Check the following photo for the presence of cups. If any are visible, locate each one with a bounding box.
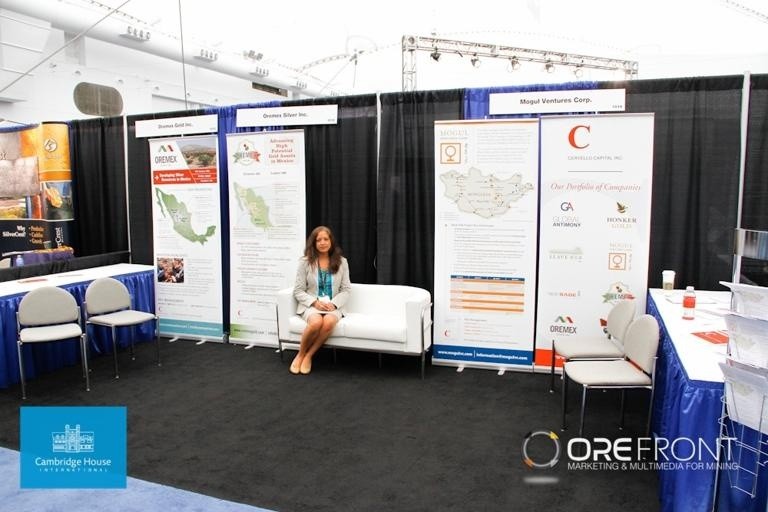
[662,270,676,296]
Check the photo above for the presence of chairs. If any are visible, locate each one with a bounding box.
[81,277,160,380]
[16,285,90,400]
[560,314,660,461]
[548,301,636,414]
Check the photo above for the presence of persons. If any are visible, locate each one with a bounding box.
[157,256,185,283]
[287,226,353,375]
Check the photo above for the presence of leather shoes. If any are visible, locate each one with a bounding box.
[300,356,312,375]
[289,353,301,375]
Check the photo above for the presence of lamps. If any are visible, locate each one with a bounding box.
[429,47,584,79]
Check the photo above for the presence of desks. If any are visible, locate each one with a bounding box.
[648,288,768,512]
[0,447,273,512]
[0,263,155,393]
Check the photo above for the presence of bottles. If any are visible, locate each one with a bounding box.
[682,285,697,320]
[16,254,24,266]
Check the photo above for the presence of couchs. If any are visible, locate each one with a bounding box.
[274,281,433,380]
